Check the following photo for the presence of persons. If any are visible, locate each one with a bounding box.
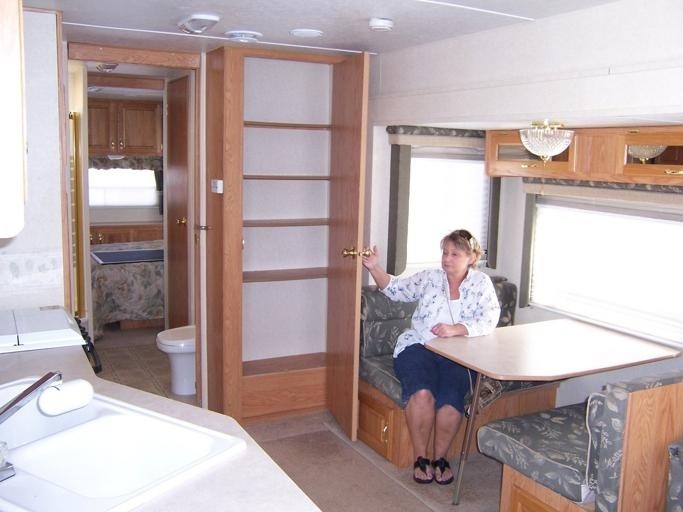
[362,229,501,485]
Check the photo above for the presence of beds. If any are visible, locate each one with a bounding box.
[91,240,167,329]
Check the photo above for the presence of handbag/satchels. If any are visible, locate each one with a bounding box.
[463,377,504,418]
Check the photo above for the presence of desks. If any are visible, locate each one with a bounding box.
[423,319,681,507]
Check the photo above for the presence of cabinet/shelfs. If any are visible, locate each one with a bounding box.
[205,46,371,442]
[88,100,163,157]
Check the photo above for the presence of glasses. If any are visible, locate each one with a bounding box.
[458,230,472,245]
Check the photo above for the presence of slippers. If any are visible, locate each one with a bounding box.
[431,457,454,486]
[413,455,435,485]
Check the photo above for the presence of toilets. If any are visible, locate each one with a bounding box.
[156,325,197,397]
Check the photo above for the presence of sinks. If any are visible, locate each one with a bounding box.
[0,376,247,512]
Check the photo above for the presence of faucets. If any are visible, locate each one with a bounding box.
[0,370,62,426]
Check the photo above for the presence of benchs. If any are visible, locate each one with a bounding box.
[359,277,560,470]
[477,367,682,512]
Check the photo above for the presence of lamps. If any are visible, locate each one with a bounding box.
[519,122,575,166]
[625,143,667,164]
